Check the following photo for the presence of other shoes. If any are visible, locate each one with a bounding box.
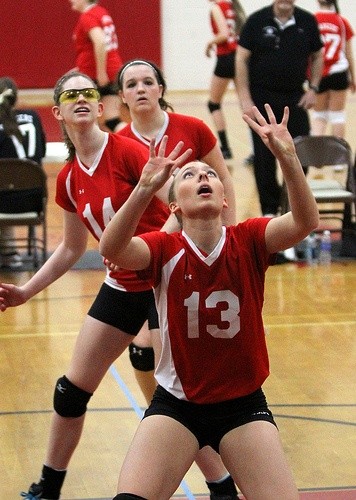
[221,149,231,160]
[20,482,60,500]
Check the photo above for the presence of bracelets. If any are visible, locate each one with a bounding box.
[308,85,319,93]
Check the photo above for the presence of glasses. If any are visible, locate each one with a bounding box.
[57,88,101,105]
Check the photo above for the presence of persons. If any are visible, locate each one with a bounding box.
[115,59,237,406]
[205,0,255,164]
[70,1,125,134]
[234,0,325,218]
[0,69,240,500]
[312,0,356,179]
[0,77,47,267]
[99,104,319,500]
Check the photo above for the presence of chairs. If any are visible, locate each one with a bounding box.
[0,159,48,269]
[282,136,356,259]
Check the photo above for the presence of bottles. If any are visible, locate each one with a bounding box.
[319,230,332,268]
